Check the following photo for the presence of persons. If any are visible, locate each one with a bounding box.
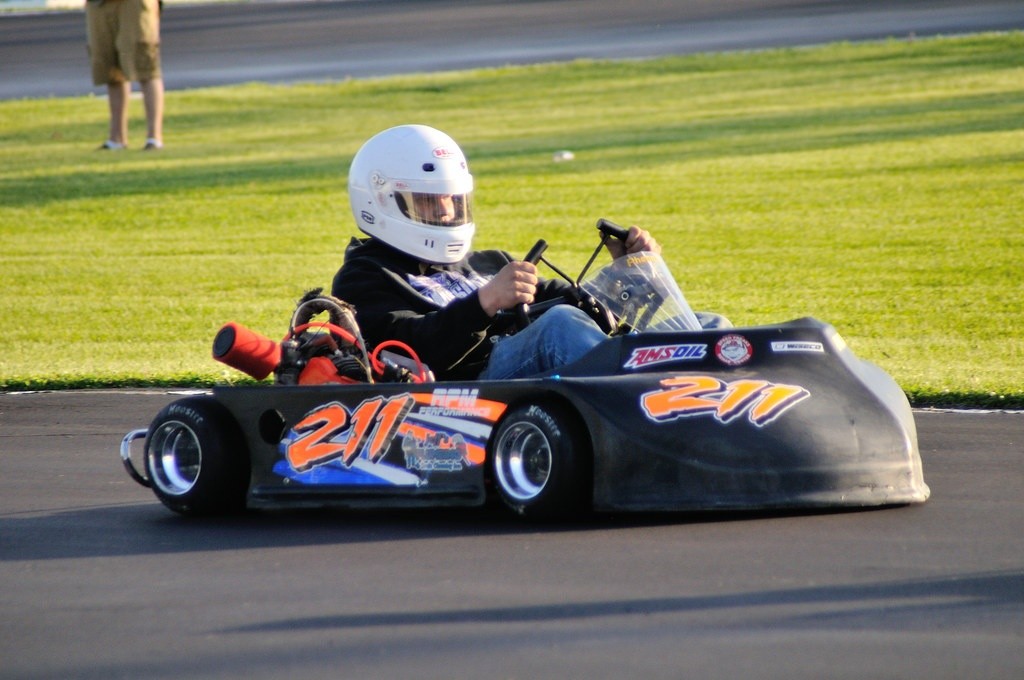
[85,0,162,150]
[329,124,663,381]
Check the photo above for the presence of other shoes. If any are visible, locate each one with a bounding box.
[91,141,124,150]
[141,139,160,150]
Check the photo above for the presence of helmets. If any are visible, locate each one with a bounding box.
[348,124,474,265]
